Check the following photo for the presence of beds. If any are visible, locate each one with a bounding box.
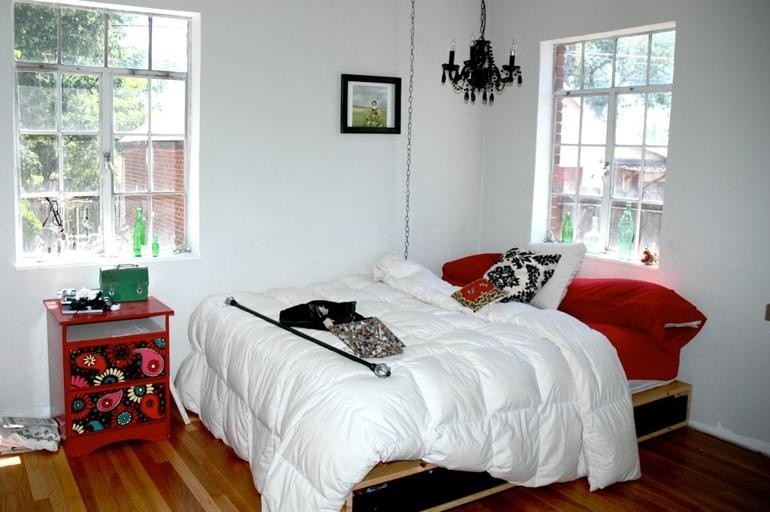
[176,275,690,511]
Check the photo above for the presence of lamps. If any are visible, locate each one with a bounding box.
[440,1,522,108]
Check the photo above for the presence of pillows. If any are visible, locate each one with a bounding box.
[483,241,587,309]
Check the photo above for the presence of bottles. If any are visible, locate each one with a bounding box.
[150,233,160,257]
[132,208,147,259]
[616,199,635,262]
[561,211,573,243]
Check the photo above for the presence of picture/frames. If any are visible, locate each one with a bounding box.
[341,73,402,134]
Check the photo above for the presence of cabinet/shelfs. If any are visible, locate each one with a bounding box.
[46,297,174,456]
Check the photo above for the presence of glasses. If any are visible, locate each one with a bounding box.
[41,196,64,230]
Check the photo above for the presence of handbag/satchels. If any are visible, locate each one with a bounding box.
[98,263,151,304]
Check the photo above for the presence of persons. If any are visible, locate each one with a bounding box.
[363,99,386,128]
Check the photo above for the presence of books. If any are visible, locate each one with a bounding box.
[59,303,106,314]
[2,415,61,428]
